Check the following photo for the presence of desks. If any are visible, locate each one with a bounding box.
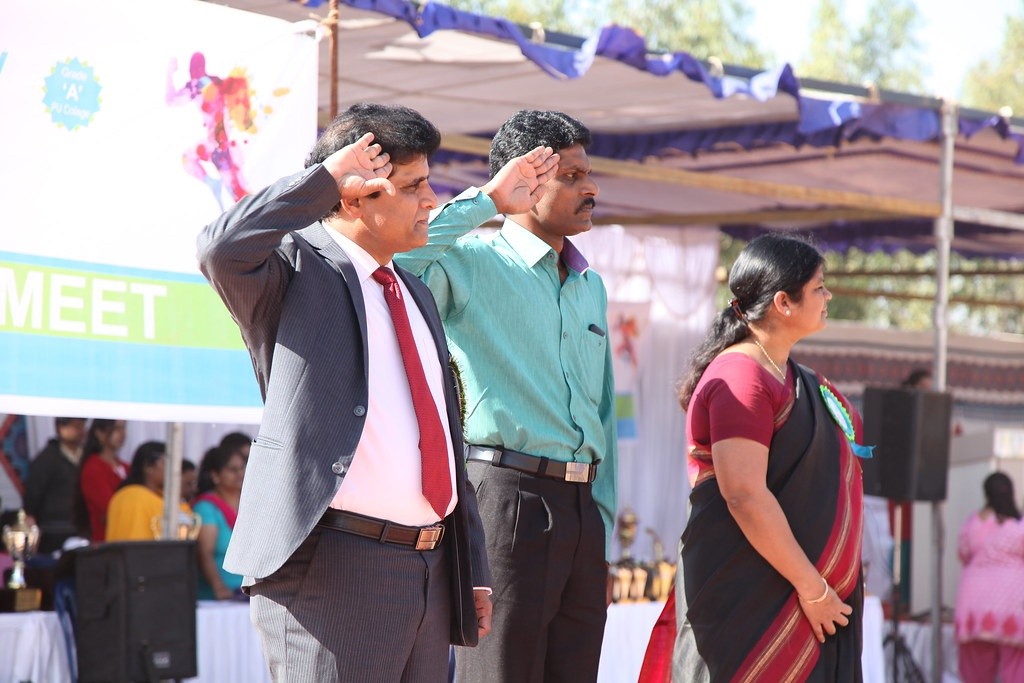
[0,593,889,682]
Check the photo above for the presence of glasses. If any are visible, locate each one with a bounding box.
[224,466,245,473]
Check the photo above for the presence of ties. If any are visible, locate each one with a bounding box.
[370,266,452,521]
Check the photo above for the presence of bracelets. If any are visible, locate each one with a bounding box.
[798,576,828,604]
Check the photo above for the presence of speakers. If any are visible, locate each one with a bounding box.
[860,387,952,503]
[68,539,200,683]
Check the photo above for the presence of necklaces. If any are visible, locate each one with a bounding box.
[750,336,786,380]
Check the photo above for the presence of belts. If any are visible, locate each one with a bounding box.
[467,445,598,483]
[316,510,445,551]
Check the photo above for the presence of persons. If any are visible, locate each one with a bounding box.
[22,418,252,602]
[672,233,877,683]
[392,110,619,683]
[952,472,1024,683]
[196,102,495,683]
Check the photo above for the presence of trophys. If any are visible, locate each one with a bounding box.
[605,514,676,603]
[0,509,42,612]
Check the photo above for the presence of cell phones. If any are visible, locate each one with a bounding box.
[588,324,606,339]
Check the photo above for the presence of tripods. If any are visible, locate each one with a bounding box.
[882,498,927,683]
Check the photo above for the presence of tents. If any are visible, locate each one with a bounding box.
[209,1,1024,682]
